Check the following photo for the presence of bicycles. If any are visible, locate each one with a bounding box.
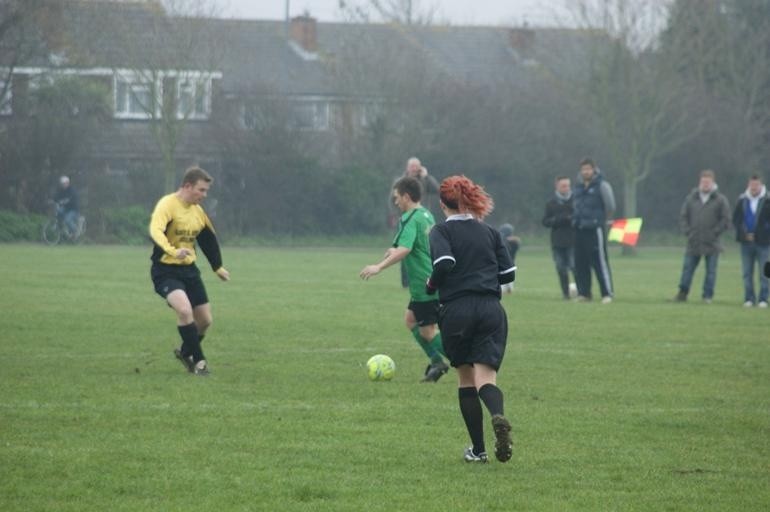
[42,201,87,245]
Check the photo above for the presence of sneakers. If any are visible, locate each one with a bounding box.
[704,296,712,304]
[493,416,513,461]
[73,230,82,241]
[675,293,691,302]
[601,295,614,304]
[192,365,212,377]
[174,347,194,373]
[758,301,769,309]
[421,360,449,384]
[742,300,755,308]
[572,294,591,303]
[463,446,488,463]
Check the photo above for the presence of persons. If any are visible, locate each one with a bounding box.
[500,223,521,295]
[424,174,517,466]
[360,176,449,384]
[388,156,440,288]
[732,175,770,308]
[148,166,230,376]
[48,176,81,245]
[675,170,731,304]
[542,176,579,301]
[571,158,616,304]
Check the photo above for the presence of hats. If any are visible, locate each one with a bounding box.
[61,176,72,184]
[499,223,513,236]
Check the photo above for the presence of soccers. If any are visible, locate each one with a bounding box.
[366,353,397,383]
